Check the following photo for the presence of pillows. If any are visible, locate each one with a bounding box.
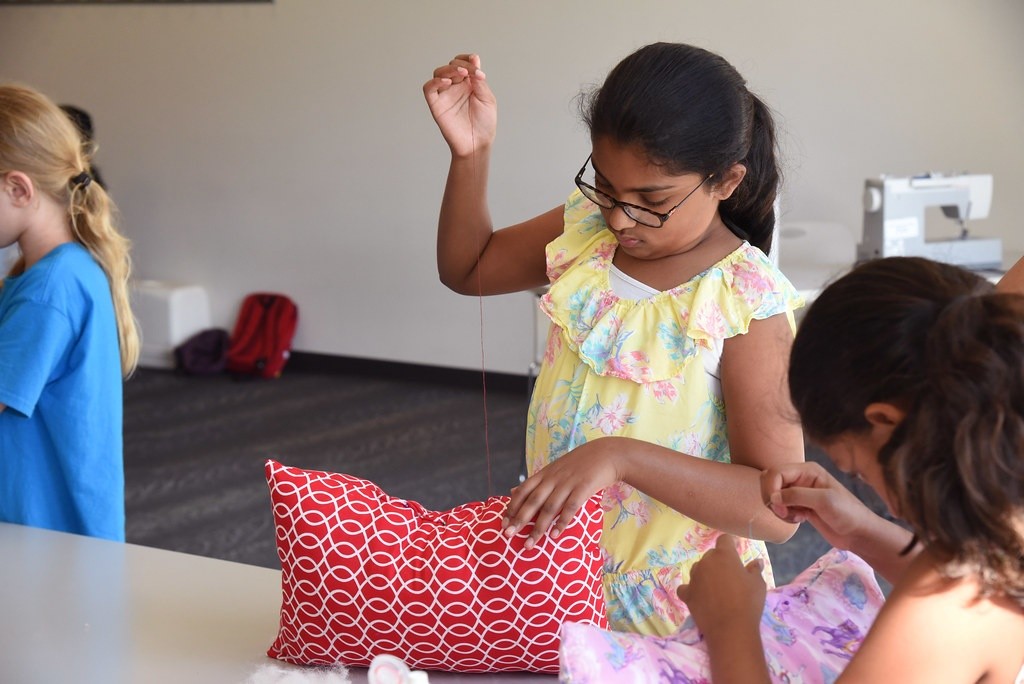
[556,549,888,683]
[262,457,608,673]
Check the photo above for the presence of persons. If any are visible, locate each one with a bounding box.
[57,103,109,191]
[0,80,144,543]
[672,254,1024,684]
[424,41,808,636]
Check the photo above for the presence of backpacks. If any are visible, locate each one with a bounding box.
[175,329,230,375]
[225,292,297,380]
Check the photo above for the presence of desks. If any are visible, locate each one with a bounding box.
[0,521,555,684]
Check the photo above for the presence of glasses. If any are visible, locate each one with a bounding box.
[574,150,719,228]
